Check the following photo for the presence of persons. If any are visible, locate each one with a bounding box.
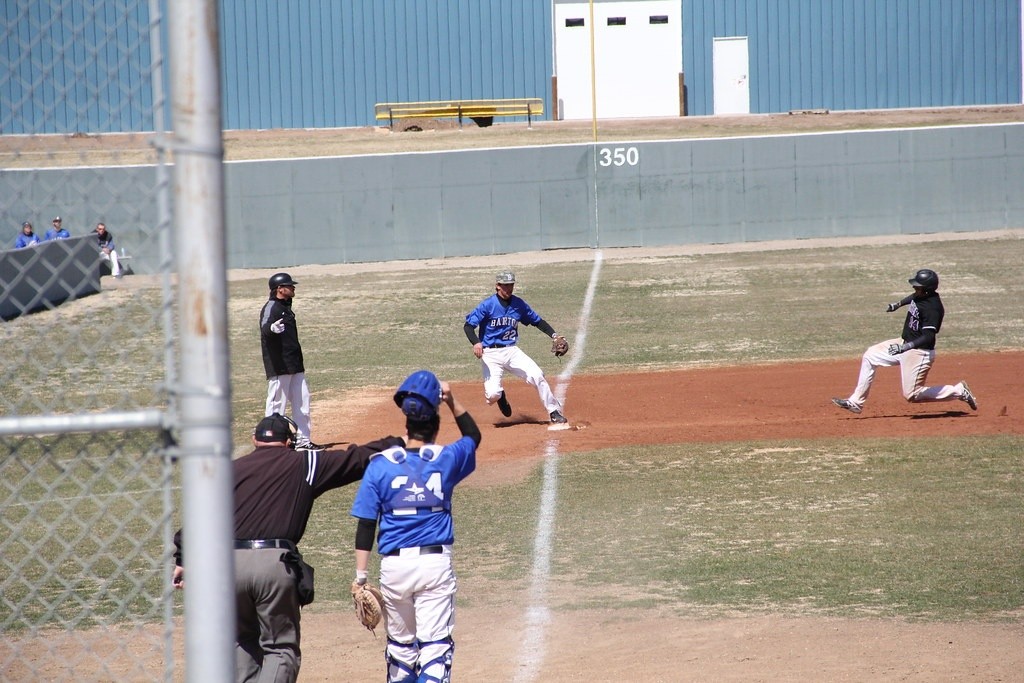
[91,223,123,278]
[463,271,569,424]
[171,412,408,683]
[832,269,978,414]
[15,222,41,249]
[351,381,481,683]
[259,273,326,450]
[44,216,70,241]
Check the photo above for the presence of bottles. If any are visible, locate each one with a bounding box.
[121,247,126,257]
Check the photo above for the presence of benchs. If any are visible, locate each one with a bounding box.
[103,255,131,273]
[375,97,543,125]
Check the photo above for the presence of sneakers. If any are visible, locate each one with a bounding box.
[832,397,862,415]
[497,390,511,417]
[550,411,567,422]
[296,442,325,452]
[959,380,977,411]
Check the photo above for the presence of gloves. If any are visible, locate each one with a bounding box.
[886,304,899,312]
[270,318,284,333]
[889,344,904,355]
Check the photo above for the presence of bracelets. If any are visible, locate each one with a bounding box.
[354,570,369,585]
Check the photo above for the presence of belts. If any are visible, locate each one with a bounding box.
[234,539,291,549]
[483,344,506,349]
[385,545,443,555]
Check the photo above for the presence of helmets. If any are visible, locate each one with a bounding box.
[269,273,298,289]
[908,269,939,290]
[394,370,439,420]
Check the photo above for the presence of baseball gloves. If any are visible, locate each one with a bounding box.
[350,578,383,640]
[551,335,569,363]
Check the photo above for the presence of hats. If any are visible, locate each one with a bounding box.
[256,413,297,442]
[53,217,61,222]
[495,270,515,284]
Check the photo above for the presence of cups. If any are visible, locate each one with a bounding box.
[101,244,109,254]
